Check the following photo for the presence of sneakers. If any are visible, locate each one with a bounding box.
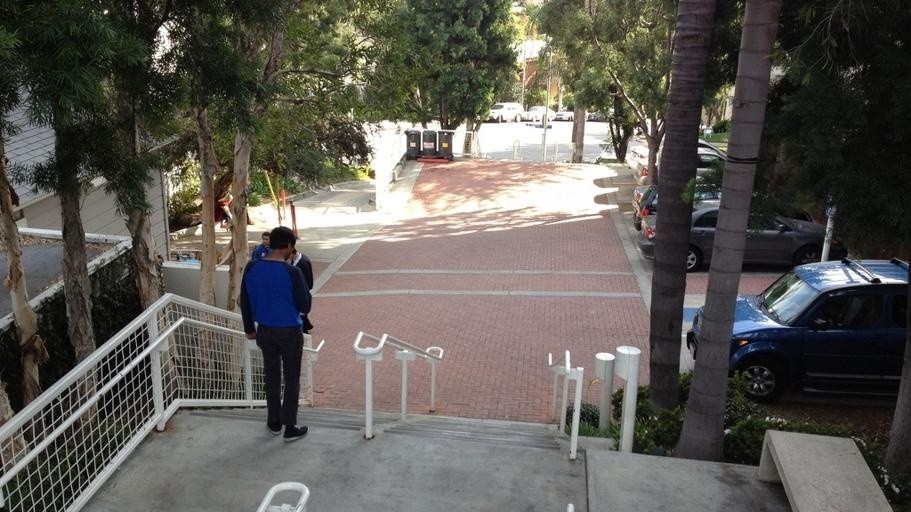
[267,422,282,434]
[284,427,308,440]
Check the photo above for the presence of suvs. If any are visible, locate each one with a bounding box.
[682,249,908,411]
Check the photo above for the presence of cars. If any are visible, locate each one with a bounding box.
[474,99,614,125]
[629,118,846,276]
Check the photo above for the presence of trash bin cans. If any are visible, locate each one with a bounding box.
[438,131,454,161]
[404,130,421,160]
[422,130,437,157]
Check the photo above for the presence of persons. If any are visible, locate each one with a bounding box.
[252,231,272,261]
[240,225,311,441]
[289,248,313,334]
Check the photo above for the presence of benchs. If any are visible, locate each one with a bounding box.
[757,423,897,512]
